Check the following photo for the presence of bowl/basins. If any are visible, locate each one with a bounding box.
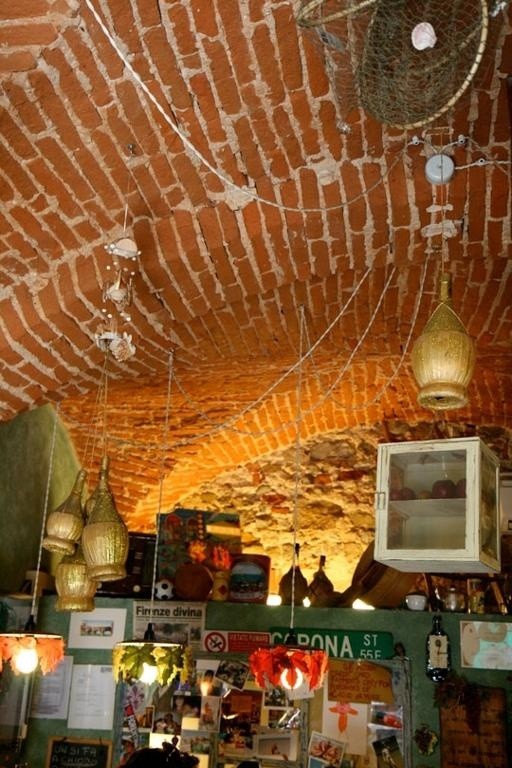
[405,593,428,611]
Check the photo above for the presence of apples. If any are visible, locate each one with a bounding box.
[390,478,466,500]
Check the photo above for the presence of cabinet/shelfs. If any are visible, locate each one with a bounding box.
[372,435,502,576]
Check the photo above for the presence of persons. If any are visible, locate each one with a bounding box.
[324,749,337,764]
[155,718,168,734]
[313,740,328,757]
[175,696,198,717]
[202,702,215,724]
[202,670,221,697]
[165,714,178,730]
[381,745,399,768]
[386,642,410,661]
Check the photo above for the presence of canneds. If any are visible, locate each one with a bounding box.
[442,587,466,612]
[467,579,486,613]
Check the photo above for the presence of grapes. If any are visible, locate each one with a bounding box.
[413,727,433,755]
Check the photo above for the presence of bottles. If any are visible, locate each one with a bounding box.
[425,614,453,684]
[278,543,334,609]
[38,453,131,616]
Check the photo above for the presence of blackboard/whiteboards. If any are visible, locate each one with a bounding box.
[44,736,114,768]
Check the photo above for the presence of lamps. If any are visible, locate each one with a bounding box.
[408,132,476,412]
